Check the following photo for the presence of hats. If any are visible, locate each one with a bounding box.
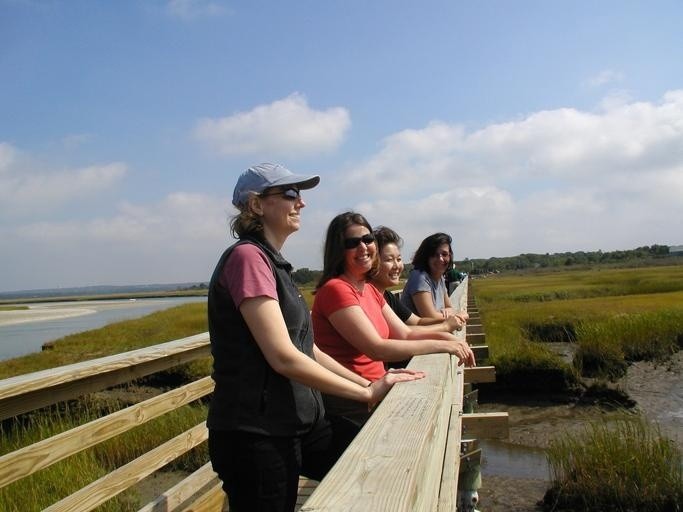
[232,162,320,207]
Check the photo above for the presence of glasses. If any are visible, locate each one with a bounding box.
[261,187,300,201]
[343,233,375,249]
